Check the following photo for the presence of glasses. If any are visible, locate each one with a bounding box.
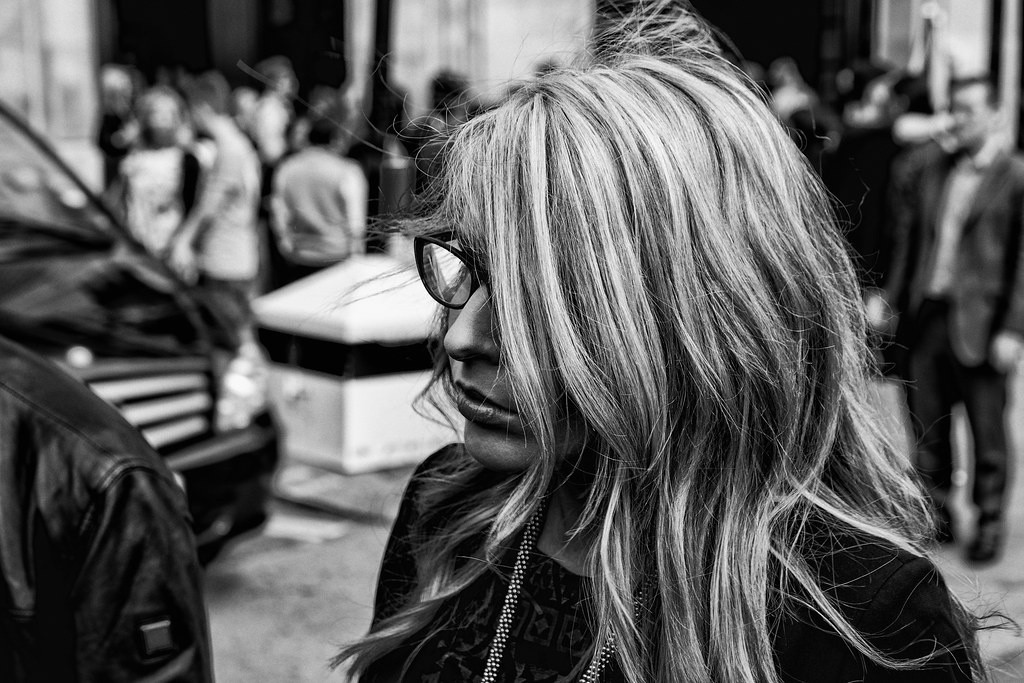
[413,223,495,311]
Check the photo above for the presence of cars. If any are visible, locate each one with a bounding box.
[0,99,288,569]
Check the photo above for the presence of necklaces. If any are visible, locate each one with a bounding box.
[481,477,712,683]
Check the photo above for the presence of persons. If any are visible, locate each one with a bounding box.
[0,336,217,683]
[328,0,1020,683]
[92,27,1024,570]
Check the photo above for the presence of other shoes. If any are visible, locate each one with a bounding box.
[969,513,1003,561]
[937,503,954,543]
